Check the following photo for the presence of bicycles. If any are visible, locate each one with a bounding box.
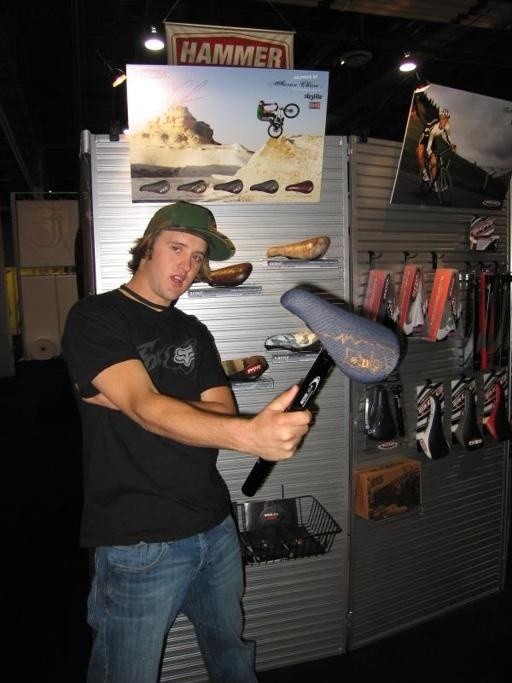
[420,145,454,207]
[268,103,299,138]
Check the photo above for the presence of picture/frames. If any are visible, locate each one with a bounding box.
[389,82,512,211]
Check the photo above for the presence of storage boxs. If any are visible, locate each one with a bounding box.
[354,457,424,522]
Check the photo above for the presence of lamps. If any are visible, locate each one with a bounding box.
[412,71,431,94]
[96,47,126,87]
[397,50,418,73]
[141,27,166,52]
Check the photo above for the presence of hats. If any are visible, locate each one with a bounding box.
[144,203,236,261]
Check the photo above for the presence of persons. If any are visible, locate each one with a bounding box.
[60,202,313,683]
[418,109,456,181]
[255,101,286,130]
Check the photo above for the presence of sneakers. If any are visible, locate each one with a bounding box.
[281,117,284,121]
[422,172,430,181]
[275,125,279,129]
[442,185,448,192]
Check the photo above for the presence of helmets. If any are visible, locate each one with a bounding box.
[259,101,264,105]
[440,109,450,118]
